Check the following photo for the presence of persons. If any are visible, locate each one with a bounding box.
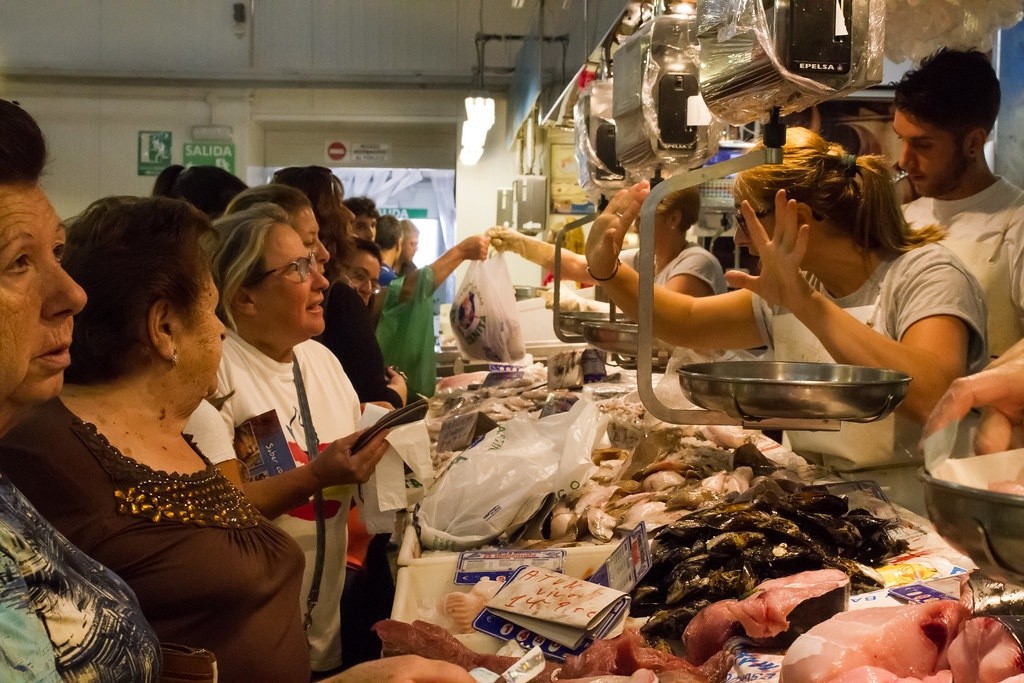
[485,185,726,298]
[0,193,314,683]
[0,98,164,683]
[585,126,991,522]
[893,48,1024,364]
[919,337,1024,455]
[272,165,492,669]
[153,164,390,683]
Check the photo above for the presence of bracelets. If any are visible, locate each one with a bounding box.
[587,258,622,281]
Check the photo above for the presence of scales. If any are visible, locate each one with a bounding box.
[637,0,915,445]
[552,0,727,370]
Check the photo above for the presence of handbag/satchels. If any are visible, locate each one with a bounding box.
[449,244,525,364]
[412,395,612,552]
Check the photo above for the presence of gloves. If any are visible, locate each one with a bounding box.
[484,225,551,268]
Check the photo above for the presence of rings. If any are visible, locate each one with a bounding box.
[615,212,622,217]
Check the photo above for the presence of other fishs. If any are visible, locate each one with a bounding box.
[414,341,791,550]
[371,567,1024,683]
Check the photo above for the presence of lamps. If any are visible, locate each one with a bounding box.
[460,32,569,165]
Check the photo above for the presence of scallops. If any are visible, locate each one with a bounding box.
[628,477,903,641]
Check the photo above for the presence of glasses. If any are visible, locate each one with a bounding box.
[243,253,318,288]
[272,164,335,195]
[348,268,381,294]
[735,207,822,240]
